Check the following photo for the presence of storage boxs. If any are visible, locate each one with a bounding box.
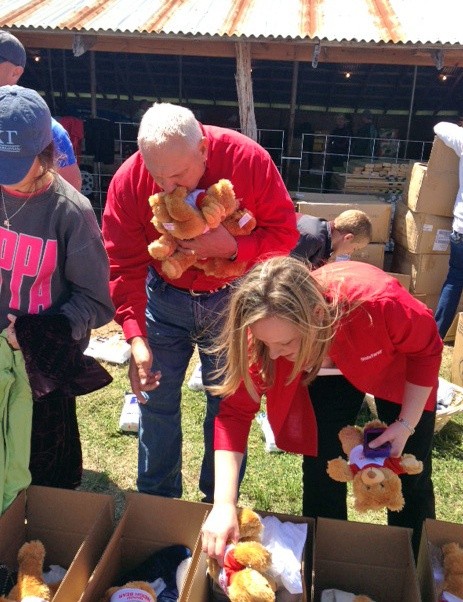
[403,131,456,216]
[392,245,451,295]
[416,294,461,341]
[1,486,116,602]
[418,518,463,602]
[392,204,454,253]
[297,203,392,243]
[316,516,421,601]
[326,244,385,268]
[185,510,317,602]
[78,493,215,602]
[451,313,462,385]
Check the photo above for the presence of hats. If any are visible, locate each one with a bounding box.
[0,30,26,67]
[0,85,52,185]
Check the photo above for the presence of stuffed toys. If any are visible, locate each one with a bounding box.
[0,538,65,602]
[206,509,278,602]
[98,581,157,602]
[161,211,256,280]
[438,540,463,601]
[145,178,240,261]
[324,419,425,514]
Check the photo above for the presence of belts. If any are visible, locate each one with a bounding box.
[151,265,231,298]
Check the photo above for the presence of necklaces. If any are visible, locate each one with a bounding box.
[1,185,37,227]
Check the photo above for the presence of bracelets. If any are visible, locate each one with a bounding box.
[399,413,416,436]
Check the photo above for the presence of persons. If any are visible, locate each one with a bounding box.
[0,31,83,191]
[326,113,352,191]
[201,257,445,568]
[292,209,373,268]
[103,99,301,506]
[0,83,115,496]
[433,119,463,341]
